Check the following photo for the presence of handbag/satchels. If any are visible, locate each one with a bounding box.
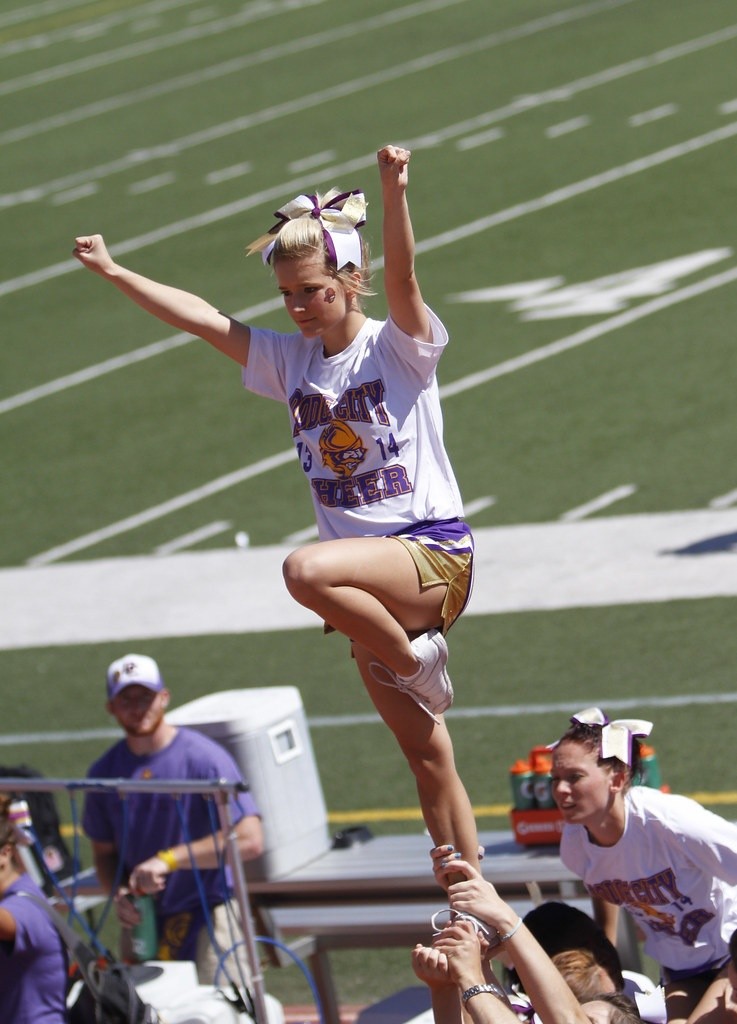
[68,944,160,1024]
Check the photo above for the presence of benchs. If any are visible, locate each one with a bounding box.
[269,898,649,1024]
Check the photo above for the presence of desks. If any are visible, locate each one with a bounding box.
[56,834,644,975]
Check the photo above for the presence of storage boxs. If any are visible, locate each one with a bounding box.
[510,807,568,846]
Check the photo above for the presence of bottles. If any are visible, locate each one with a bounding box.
[510,747,556,809]
[119,877,158,964]
[634,743,659,788]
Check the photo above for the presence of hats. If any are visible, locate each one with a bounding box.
[107,654,162,699]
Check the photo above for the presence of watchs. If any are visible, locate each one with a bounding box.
[462,983,512,1013]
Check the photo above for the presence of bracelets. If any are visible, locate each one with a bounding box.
[155,849,176,872]
[502,917,525,942]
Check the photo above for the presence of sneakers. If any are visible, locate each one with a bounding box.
[427,912,500,956]
[399,629,454,714]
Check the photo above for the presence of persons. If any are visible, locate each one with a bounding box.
[81,653,262,991]
[71,145,503,961]
[411,705,737,1024]
[0,816,70,1024]
